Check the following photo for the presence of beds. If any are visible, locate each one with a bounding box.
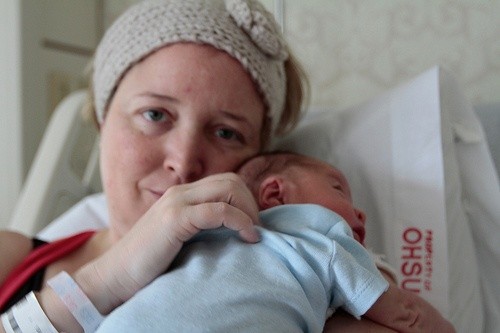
[7,87,499,238]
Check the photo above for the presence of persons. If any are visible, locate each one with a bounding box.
[0,0,415,333]
[97,149,459,333]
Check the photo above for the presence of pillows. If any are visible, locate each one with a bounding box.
[36,65,498,333]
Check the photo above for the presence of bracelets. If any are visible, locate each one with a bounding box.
[0,290,60,333]
[47,271,105,333]
